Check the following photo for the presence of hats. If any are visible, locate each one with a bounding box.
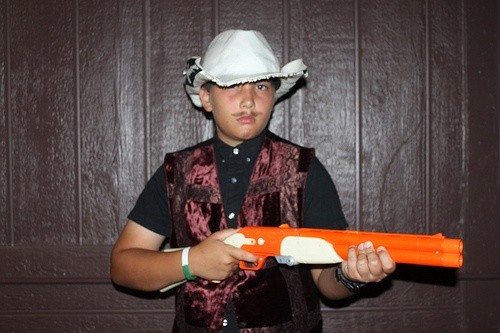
[182,28,308,107]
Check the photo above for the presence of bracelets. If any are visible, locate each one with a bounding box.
[182,246,198,281]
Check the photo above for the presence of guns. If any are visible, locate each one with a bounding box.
[210,223,463,283]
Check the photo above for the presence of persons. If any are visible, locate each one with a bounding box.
[110,28,396,333]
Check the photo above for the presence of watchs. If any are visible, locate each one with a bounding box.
[336,263,366,291]
